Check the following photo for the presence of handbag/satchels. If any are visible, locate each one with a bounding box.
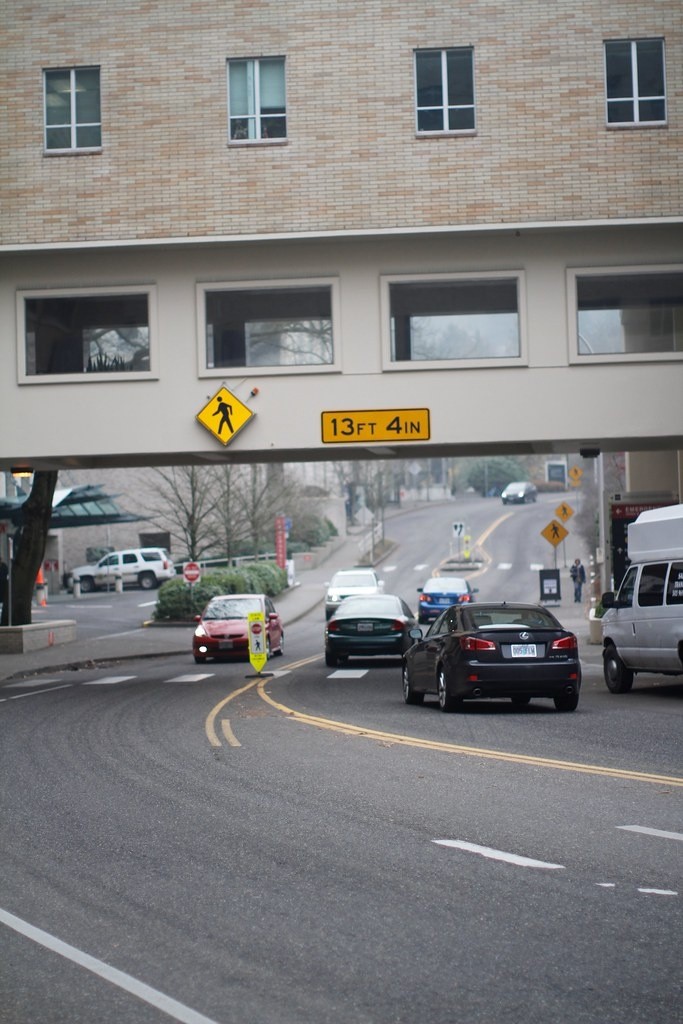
[572,572,577,577]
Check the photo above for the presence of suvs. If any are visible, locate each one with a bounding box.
[69,548,176,594]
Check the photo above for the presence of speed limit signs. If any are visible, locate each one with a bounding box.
[183,562,201,583]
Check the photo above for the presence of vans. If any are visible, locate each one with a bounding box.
[601,504,683,694]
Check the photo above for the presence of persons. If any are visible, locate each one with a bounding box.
[570,557,586,602]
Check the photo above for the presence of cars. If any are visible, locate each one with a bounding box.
[324,594,421,667]
[192,594,285,664]
[418,577,480,624]
[401,602,582,713]
[501,481,538,505]
[322,568,386,622]
